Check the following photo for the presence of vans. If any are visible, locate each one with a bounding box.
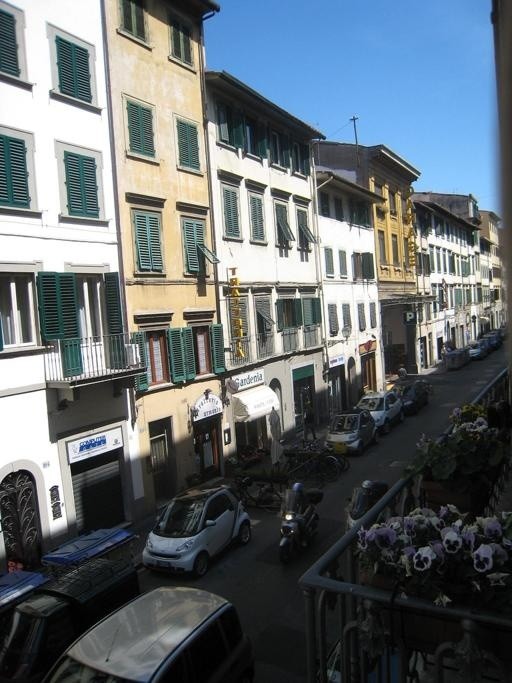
[45,582,259,682]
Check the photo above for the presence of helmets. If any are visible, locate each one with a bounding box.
[362,480,372,489]
[292,483,302,491]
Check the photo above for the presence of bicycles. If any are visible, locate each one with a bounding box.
[272,438,354,492]
[229,473,281,515]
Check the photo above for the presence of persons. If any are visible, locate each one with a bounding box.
[301,400,318,440]
[397,362,408,379]
[280,482,314,549]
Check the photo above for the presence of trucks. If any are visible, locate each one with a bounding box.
[5,556,141,683]
[0,526,143,639]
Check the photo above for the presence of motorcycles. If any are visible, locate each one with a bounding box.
[278,488,325,564]
[342,482,389,536]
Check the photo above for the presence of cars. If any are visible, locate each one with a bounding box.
[352,390,404,436]
[323,408,380,458]
[386,377,434,416]
[140,483,253,580]
[465,325,506,361]
